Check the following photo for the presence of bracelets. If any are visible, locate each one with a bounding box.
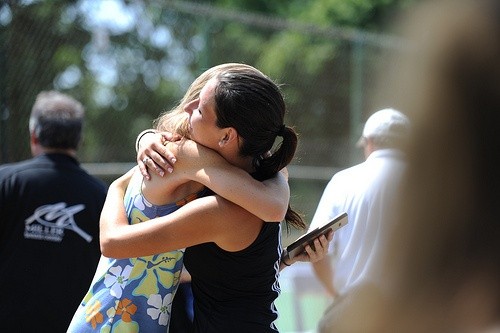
[136,128,157,151]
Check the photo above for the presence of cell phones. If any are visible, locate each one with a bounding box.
[287,213,348,260]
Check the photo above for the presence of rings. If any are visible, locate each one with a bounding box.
[143,156,150,163]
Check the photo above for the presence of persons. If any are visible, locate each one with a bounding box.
[304,107,412,332]
[99,62,306,333]
[66,62,335,332]
[0,89,108,333]
[317,0,499,333]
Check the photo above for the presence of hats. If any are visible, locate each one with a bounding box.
[356,109,409,148]
